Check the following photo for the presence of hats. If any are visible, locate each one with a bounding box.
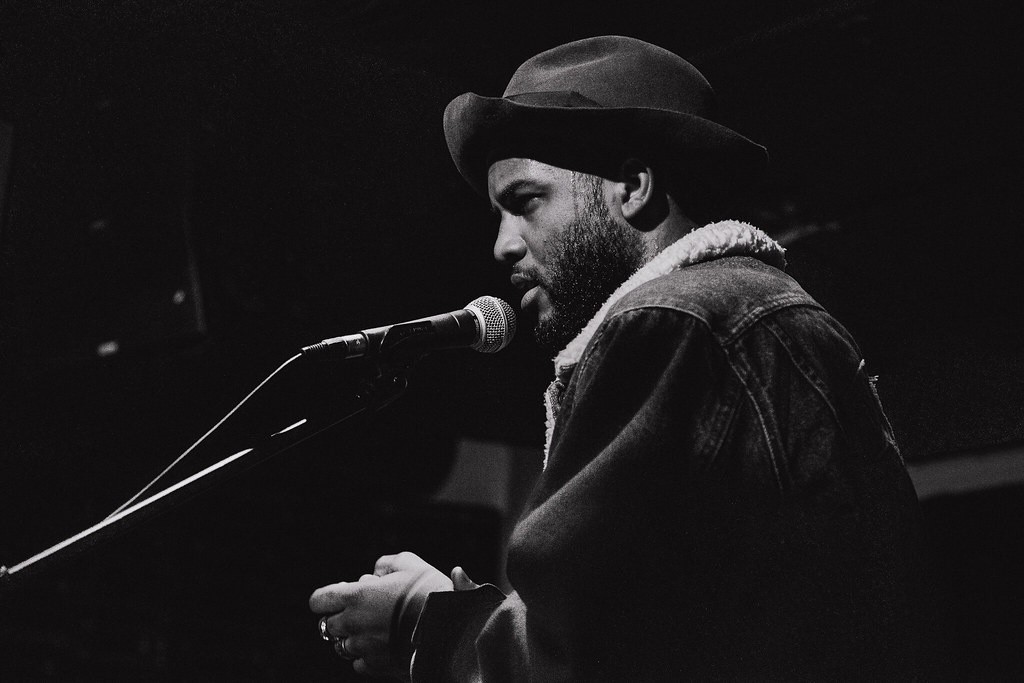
[443,35,769,203]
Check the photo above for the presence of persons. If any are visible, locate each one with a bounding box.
[309,35,965,682]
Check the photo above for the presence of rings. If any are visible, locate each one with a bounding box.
[341,638,348,654]
[353,655,369,674]
[318,615,339,642]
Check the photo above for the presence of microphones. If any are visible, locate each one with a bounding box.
[300,294,517,364]
